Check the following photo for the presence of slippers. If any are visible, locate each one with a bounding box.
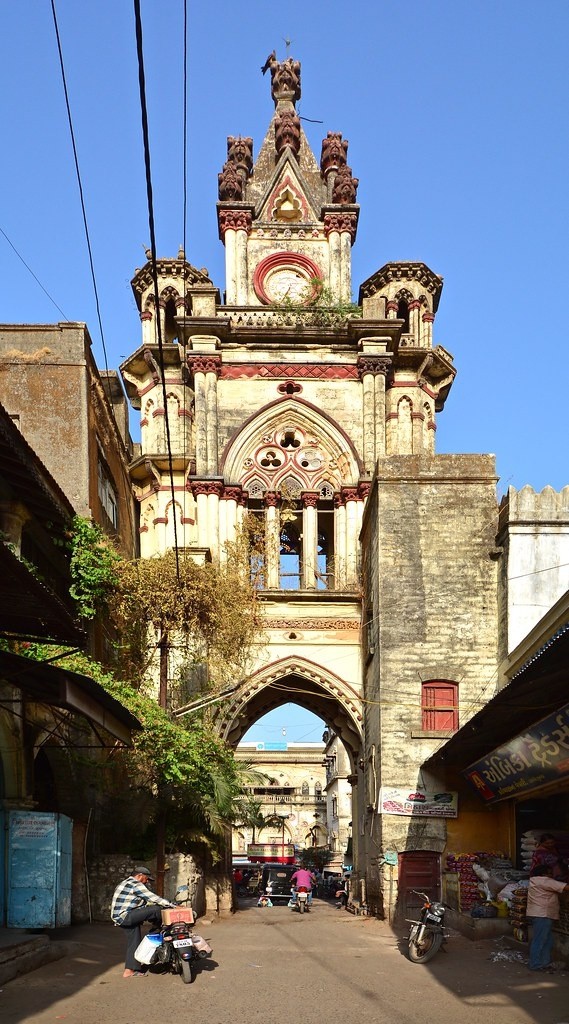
[127,971,148,977]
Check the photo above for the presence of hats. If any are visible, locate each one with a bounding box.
[134,867,157,881]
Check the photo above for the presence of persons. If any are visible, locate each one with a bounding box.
[526,864,569,974]
[233,865,349,905]
[528,834,569,883]
[111,867,185,978]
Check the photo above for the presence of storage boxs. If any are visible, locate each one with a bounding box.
[161,908,194,925]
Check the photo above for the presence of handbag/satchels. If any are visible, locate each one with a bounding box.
[471,898,509,918]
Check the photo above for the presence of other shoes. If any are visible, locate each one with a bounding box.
[340,905,347,910]
[532,966,557,974]
[291,899,297,904]
[335,902,342,906]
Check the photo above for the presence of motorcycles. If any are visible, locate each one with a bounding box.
[289,881,318,914]
[155,885,199,983]
[403,890,455,964]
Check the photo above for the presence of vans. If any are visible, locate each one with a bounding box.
[237,864,297,902]
